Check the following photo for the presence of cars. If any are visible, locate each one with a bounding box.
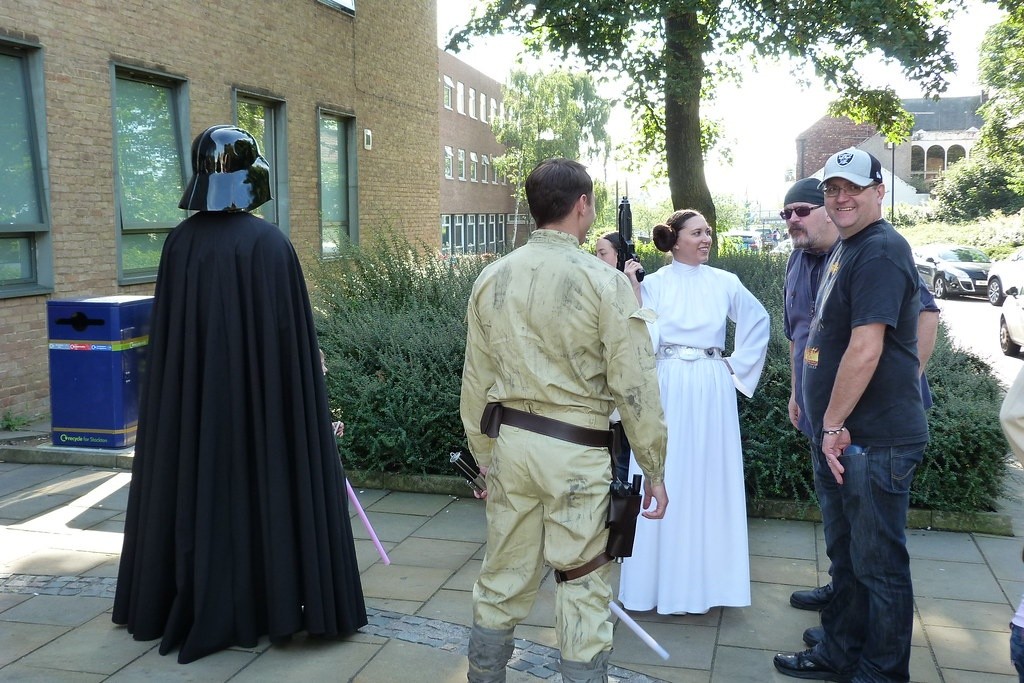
[728,227,778,251]
[910,244,993,299]
[986,247,1024,357]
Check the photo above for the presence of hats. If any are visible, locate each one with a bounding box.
[817,147,882,190]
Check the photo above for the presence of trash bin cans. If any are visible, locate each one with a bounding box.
[46,295,156,453]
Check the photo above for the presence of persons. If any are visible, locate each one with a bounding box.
[782,176,940,647]
[459,159,670,683]
[617,208,771,615]
[773,145,930,683]
[595,230,618,267]
[110,122,369,665]
[999,362,1024,682]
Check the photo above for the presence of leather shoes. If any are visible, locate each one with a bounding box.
[773,648,852,682]
[803,626,824,645]
[790,585,834,609]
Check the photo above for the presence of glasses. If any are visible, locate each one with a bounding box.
[780,205,822,219]
[823,184,878,197]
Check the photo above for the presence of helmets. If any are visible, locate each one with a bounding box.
[178,124,271,212]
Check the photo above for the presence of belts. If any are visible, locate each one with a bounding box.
[656,345,723,360]
[501,407,612,447]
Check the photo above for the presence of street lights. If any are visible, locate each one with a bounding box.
[884,141,895,226]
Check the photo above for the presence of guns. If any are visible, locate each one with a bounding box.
[616,197,645,283]
[610,480,633,564]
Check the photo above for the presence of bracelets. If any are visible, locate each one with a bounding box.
[820,427,845,435]
